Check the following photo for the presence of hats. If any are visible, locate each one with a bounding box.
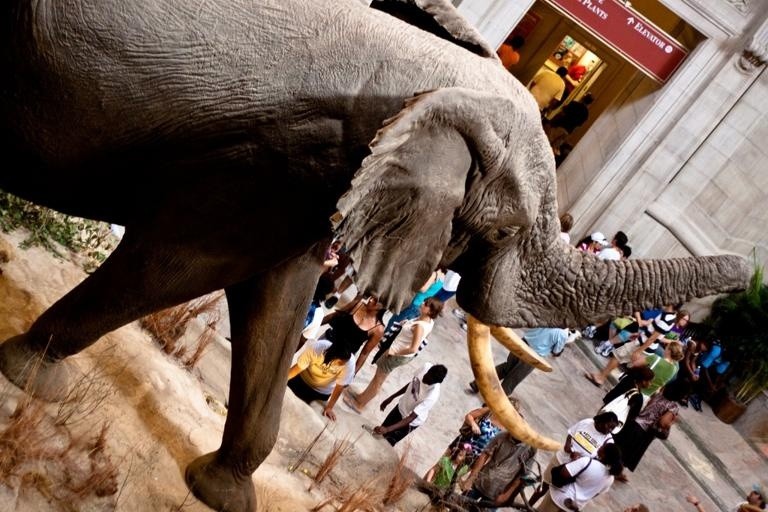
[753,483,768,503]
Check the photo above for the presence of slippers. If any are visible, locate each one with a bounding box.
[585,372,601,388]
[343,388,361,414]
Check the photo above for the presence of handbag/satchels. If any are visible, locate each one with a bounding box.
[599,389,640,435]
[460,416,481,437]
[552,463,576,487]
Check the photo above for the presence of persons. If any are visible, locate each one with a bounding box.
[497,36,525,70]
[546,94,594,149]
[529,66,568,116]
[569,65,592,81]
[288,213,767,511]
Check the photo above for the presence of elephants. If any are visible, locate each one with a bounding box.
[0,1,756,512]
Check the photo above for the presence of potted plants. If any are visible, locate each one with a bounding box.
[712,244,767,425]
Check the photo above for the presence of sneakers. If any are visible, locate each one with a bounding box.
[566,330,582,344]
[690,397,702,412]
[452,308,467,322]
[460,323,467,332]
[595,340,615,357]
[582,325,597,339]
